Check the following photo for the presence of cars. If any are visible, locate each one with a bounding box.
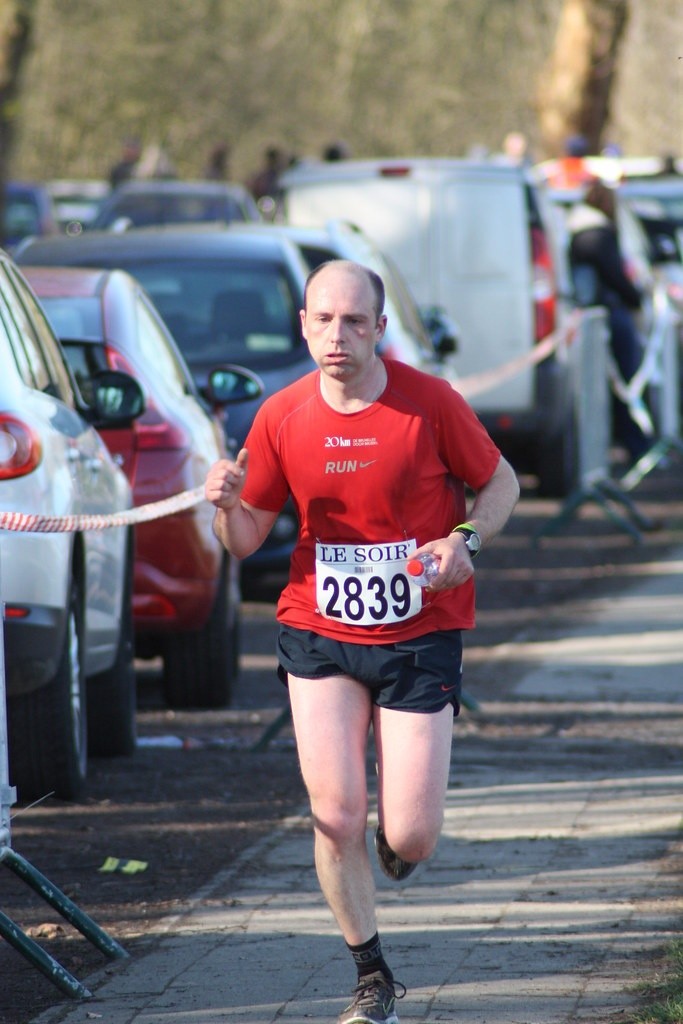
[15,268,262,710]
[0,251,132,798]
[0,154,683,597]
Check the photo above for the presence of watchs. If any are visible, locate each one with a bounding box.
[454,528,481,558]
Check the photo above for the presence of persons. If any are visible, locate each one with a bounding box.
[205,258,520,1024]
[567,181,671,470]
[503,133,586,166]
[203,146,230,181]
[249,146,293,198]
[112,141,178,190]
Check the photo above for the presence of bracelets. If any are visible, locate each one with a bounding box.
[452,524,480,559]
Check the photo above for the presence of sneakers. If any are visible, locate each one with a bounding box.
[373,826,419,882]
[337,971,407,1024]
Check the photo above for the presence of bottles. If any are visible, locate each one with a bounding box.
[407,553,442,587]
